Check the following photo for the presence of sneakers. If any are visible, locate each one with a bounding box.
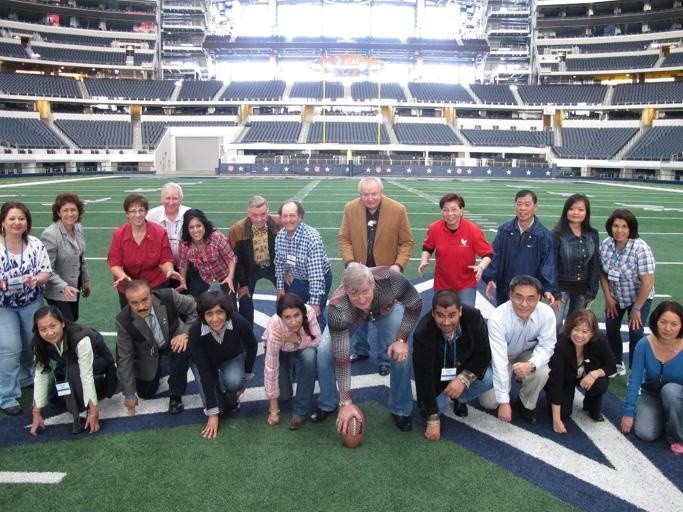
[607,361,626,378]
[670,443,682,454]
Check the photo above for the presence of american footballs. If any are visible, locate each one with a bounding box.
[339,404,366,449]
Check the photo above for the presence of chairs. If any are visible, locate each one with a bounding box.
[0,1,682,175]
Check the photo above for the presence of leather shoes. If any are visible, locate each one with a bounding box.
[309,408,337,423]
[520,406,539,425]
[582,400,603,421]
[288,416,306,430]
[378,365,389,376]
[451,399,467,417]
[390,412,412,431]
[223,390,237,409]
[349,353,369,363]
[169,400,183,414]
[4,405,22,416]
[71,416,86,434]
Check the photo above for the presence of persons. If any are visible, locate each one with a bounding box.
[108,180,332,439]
[1,193,124,434]
[319,176,682,455]
